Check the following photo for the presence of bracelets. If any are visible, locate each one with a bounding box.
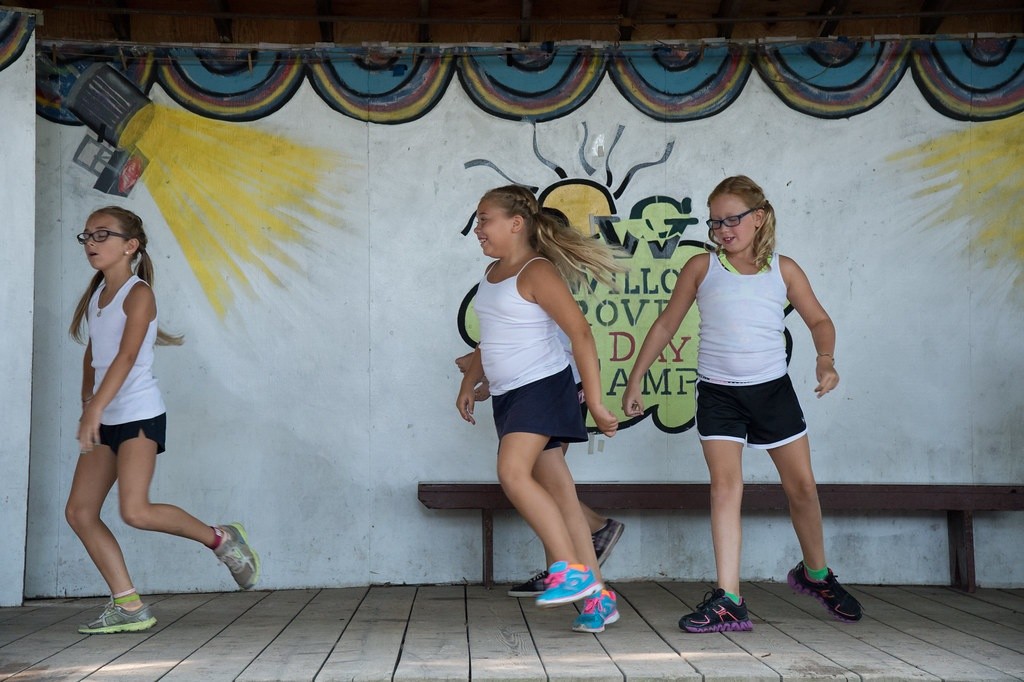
[81,394,94,407]
[816,352,835,366]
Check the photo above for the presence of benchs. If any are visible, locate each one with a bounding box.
[417,482,1024,594]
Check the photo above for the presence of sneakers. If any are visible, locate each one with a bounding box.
[786,560,862,623]
[509,570,550,598]
[212,521,260,590]
[678,586,754,634]
[535,559,604,609]
[571,589,621,634]
[78,596,158,632]
[590,518,624,569]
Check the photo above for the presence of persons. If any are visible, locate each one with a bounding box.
[453,204,628,599]
[624,173,863,634]
[65,205,259,636]
[456,183,622,634]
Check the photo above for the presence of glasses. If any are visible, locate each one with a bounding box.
[76,230,130,244]
[706,208,757,231]
